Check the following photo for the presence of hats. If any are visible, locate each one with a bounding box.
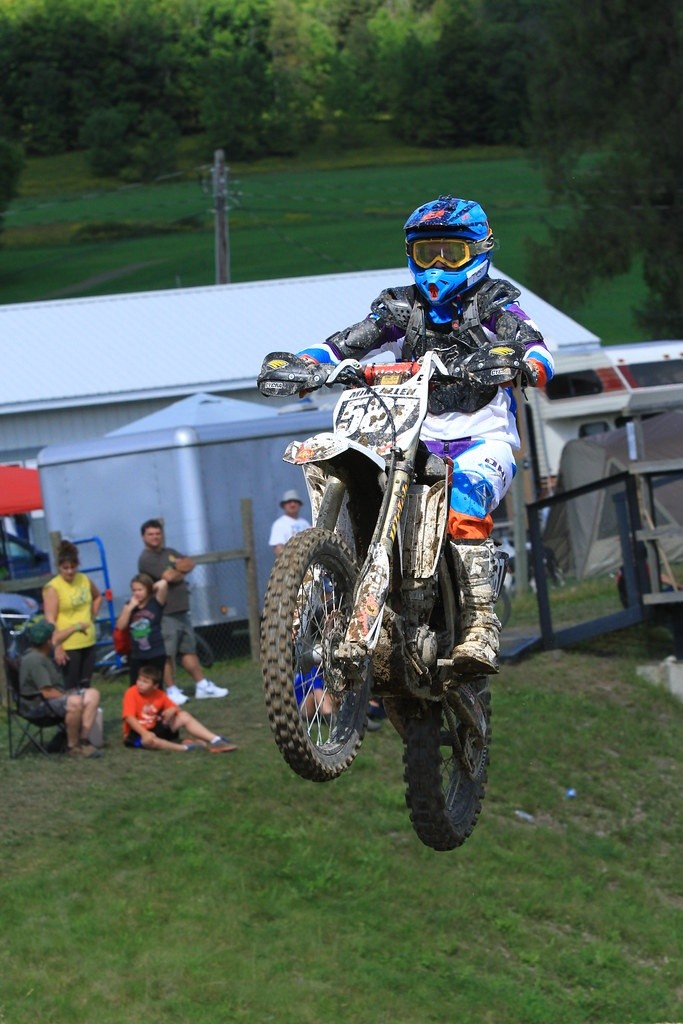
[280,490,303,507]
[30,622,55,644]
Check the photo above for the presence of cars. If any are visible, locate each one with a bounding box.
[0,529,51,679]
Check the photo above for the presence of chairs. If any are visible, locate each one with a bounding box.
[3,655,64,759]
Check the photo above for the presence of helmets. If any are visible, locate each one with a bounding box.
[403,194,494,307]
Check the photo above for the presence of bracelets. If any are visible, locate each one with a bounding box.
[75,624,81,632]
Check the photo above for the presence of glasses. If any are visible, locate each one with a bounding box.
[405,234,494,269]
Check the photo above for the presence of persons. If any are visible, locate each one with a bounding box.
[118,574,167,688]
[121,667,238,752]
[18,619,101,760]
[139,519,230,705]
[295,607,388,731]
[262,195,554,674]
[41,540,103,690]
[269,489,311,557]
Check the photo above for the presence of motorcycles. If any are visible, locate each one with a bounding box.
[256,346,513,854]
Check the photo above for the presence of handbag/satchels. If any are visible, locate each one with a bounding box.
[113,627,131,655]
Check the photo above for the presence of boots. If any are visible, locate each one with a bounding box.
[450,541,501,674]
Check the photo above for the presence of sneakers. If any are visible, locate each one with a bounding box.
[207,738,237,752]
[366,717,382,730]
[195,678,229,699]
[166,685,190,704]
[181,738,208,751]
[69,744,99,757]
